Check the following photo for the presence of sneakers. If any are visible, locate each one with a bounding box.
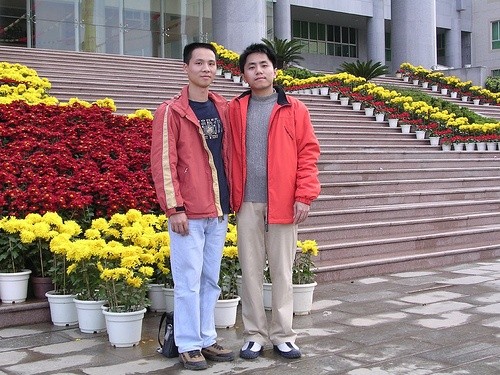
[200,343,234,362]
[179,350,208,370]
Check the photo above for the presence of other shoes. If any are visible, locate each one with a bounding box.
[240,339,263,359]
[273,340,301,358]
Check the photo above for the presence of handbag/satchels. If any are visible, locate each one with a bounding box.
[158,311,178,357]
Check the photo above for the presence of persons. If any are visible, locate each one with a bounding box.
[173,44,320,359]
[150,43,230,370]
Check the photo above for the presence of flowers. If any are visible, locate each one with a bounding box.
[0,41,500,311]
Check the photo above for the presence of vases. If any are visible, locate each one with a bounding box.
[329,93,340,100]
[1,269,32,304]
[453,142,464,151]
[429,137,440,145]
[363,107,375,116]
[442,143,451,151]
[339,97,349,105]
[352,103,361,110]
[415,131,426,139]
[399,124,412,133]
[487,142,497,151]
[311,88,320,95]
[388,118,399,127]
[320,87,329,96]
[476,142,487,151]
[35,276,317,348]
[465,142,475,151]
[375,113,384,121]
[304,89,311,94]
